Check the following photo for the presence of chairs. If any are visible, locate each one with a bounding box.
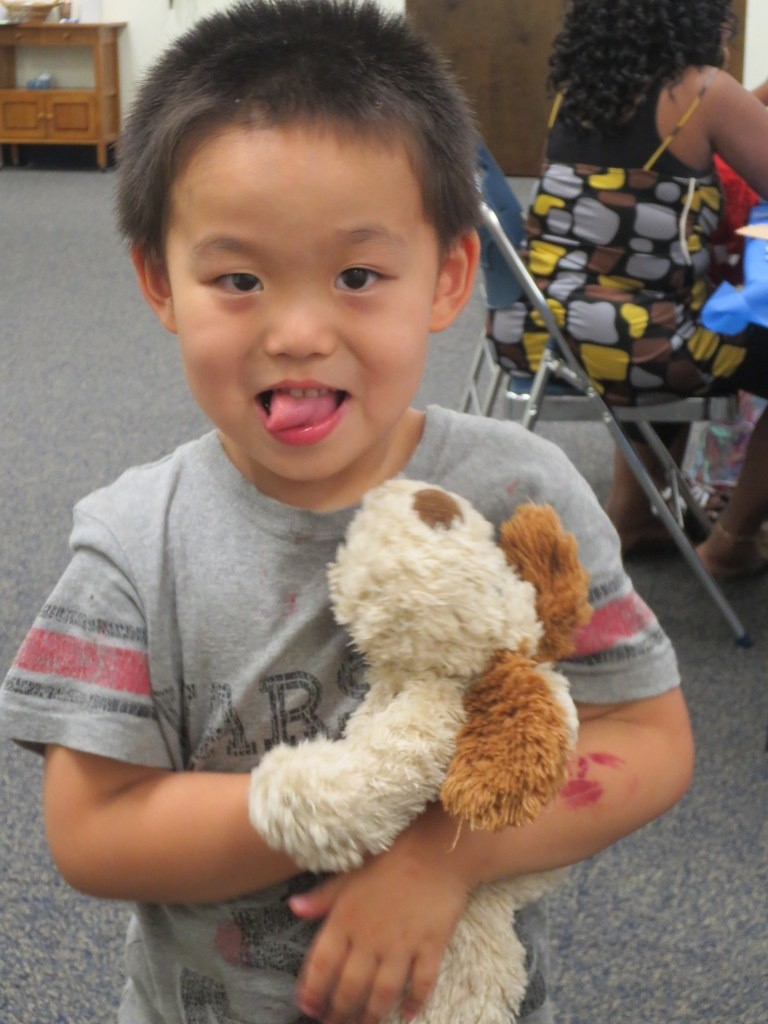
[453,134,748,647]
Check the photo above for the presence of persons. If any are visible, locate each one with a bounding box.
[485,0,768,581]
[0,0,695,1024]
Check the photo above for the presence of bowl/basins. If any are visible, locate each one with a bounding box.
[0,0,61,24]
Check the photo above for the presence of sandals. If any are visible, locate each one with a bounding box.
[698,520,768,582]
[645,490,689,544]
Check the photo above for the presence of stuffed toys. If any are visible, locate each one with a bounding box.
[248,472,593,1024]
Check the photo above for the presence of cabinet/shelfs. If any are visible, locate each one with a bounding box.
[0,21,128,170]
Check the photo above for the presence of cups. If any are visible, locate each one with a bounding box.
[58,0,71,18]
